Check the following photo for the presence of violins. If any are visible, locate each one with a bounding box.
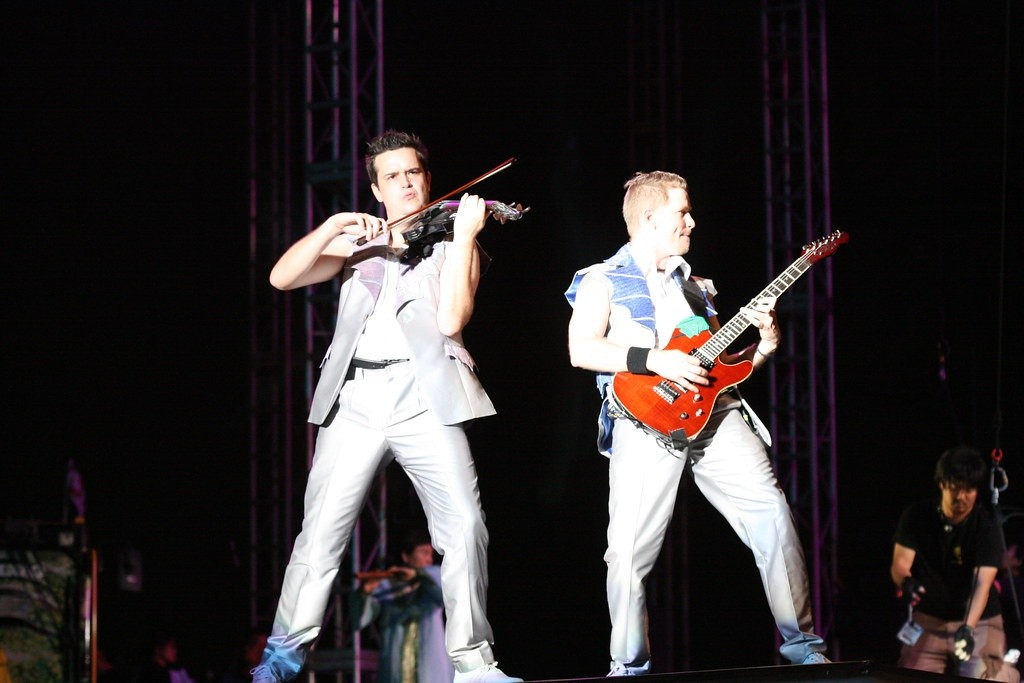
[400,194,526,246]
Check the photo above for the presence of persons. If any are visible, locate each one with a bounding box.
[0,496,86,683]
[347,538,444,683]
[250,130,524,683]
[891,447,1004,682]
[98,625,270,683]
[565,171,832,677]
[998,543,1024,667]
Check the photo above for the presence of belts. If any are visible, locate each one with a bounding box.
[351,358,410,369]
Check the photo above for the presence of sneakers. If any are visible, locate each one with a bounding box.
[802,652,832,664]
[606,659,651,676]
[453,661,524,683]
[250,664,281,683]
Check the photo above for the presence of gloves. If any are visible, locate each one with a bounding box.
[895,577,925,606]
[953,624,975,661]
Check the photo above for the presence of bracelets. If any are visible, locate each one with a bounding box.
[625,346,650,375]
[757,348,769,358]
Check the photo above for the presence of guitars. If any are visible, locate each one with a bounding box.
[614,229,849,449]
